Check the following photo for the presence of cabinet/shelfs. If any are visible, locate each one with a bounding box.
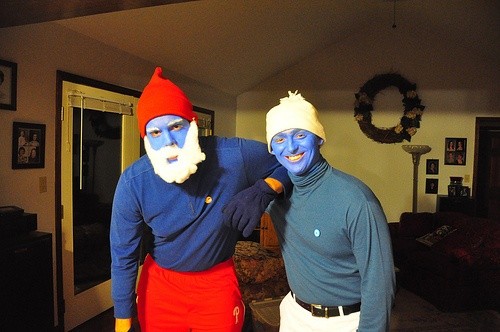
[0,233,53,332]
[437,195,472,214]
[472,117,500,219]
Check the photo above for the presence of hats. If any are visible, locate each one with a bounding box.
[265,89,327,154]
[136,67,198,140]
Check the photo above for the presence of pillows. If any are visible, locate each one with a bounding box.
[233,242,282,282]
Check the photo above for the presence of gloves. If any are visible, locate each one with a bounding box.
[220,177,279,237]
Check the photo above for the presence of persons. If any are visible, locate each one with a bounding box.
[263,90,396,332]
[110,67,294,332]
[17,129,40,168]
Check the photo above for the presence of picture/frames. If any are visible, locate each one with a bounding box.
[0,59,18,110]
[444,138,467,165]
[426,159,439,174]
[12,122,46,168]
[425,178,438,194]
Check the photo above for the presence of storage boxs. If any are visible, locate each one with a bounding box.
[249,298,283,332]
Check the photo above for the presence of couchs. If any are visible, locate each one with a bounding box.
[241,274,290,305]
[388,212,500,313]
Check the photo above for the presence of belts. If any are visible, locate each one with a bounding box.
[290,291,360,318]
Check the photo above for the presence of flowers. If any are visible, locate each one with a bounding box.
[353,73,426,144]
